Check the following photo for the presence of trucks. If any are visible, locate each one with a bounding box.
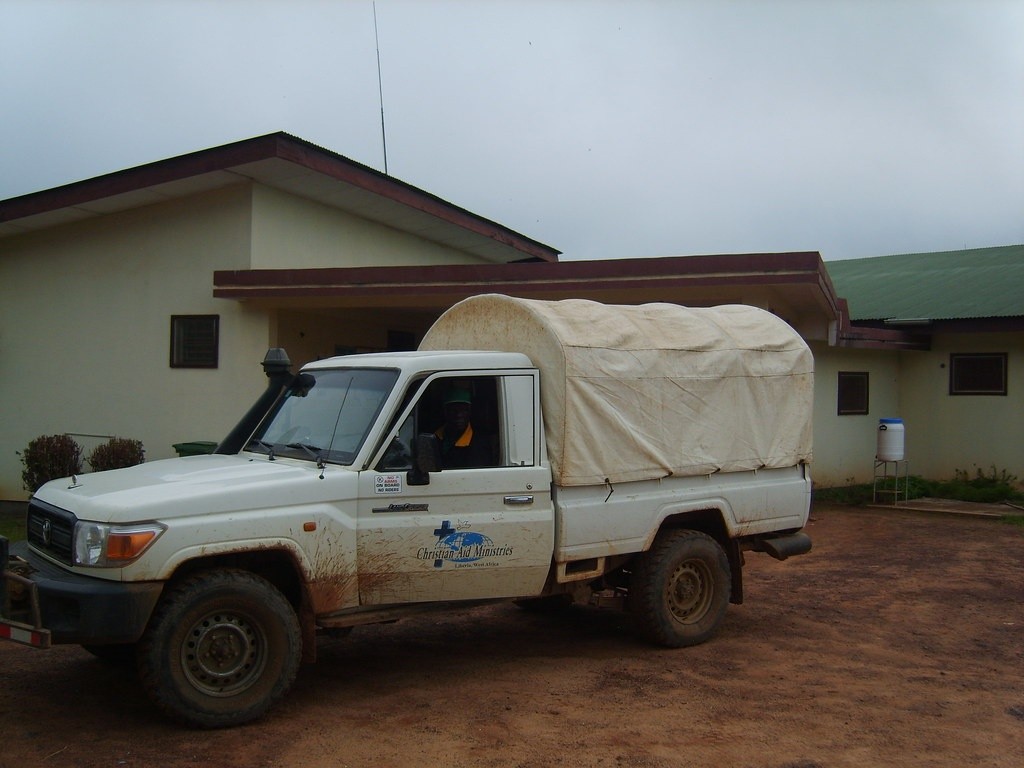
[0,291,817,728]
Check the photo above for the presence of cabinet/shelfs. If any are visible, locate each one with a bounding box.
[873,459,909,507]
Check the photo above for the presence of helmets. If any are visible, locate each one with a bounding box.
[444,387,472,404]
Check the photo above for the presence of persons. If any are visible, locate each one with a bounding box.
[431,383,495,470]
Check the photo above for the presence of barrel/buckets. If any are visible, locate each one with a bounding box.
[877,418,905,462]
